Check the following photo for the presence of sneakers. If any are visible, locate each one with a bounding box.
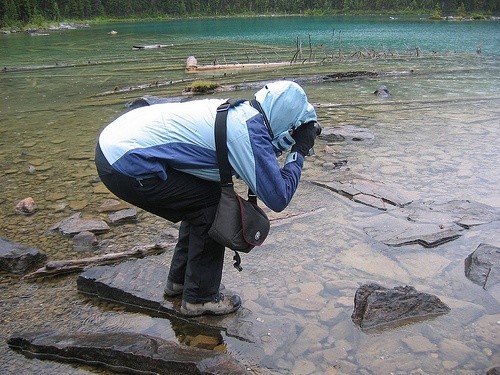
[180,292,241,317]
[163,279,225,297]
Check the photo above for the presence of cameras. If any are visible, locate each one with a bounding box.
[293,122,322,142]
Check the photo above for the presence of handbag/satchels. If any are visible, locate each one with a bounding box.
[208,186,270,254]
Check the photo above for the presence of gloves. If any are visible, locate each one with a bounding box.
[291,124,315,157]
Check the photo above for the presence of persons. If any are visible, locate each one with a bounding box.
[96,80,317,316]
